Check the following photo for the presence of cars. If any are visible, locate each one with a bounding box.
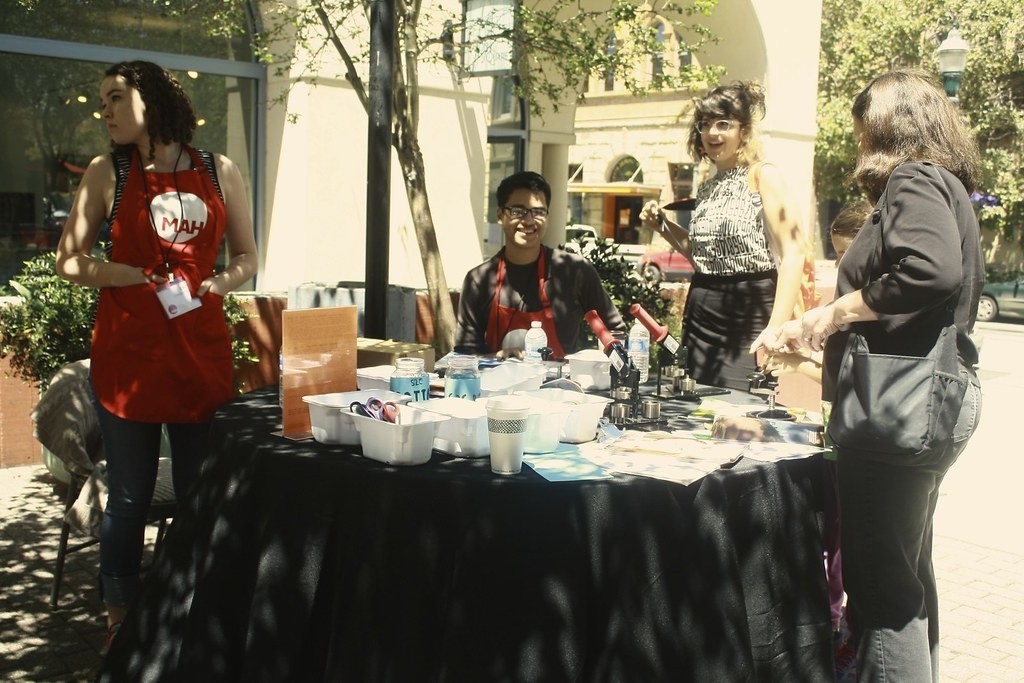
[975,274,1024,322]
[636,243,696,283]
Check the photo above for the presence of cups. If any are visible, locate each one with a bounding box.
[488,395,530,474]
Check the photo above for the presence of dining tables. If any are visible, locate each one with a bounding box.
[103,367,842,683]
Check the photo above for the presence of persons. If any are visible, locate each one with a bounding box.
[454,171,630,361]
[639,79,806,392]
[771,200,875,413]
[770,68,983,683]
[55,59,259,656]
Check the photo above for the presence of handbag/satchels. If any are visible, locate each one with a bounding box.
[61,458,108,540]
[827,324,970,469]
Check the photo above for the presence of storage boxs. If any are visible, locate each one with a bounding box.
[301,337,615,467]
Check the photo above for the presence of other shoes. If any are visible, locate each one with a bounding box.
[834,641,856,680]
[107,620,123,649]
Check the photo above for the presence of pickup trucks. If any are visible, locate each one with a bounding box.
[564,221,644,270]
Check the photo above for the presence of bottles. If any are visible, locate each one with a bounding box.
[627,317,650,384]
[524,320,547,366]
[391,357,430,402]
[444,354,480,400]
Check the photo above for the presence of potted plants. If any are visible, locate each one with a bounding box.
[0,237,106,485]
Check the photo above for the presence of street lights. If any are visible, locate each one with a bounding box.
[933,26,971,107]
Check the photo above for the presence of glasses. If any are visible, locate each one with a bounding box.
[692,118,749,134]
[499,203,550,219]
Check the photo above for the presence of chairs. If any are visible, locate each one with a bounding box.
[30,358,177,610]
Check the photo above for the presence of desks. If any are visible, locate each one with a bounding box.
[294,282,461,347]
